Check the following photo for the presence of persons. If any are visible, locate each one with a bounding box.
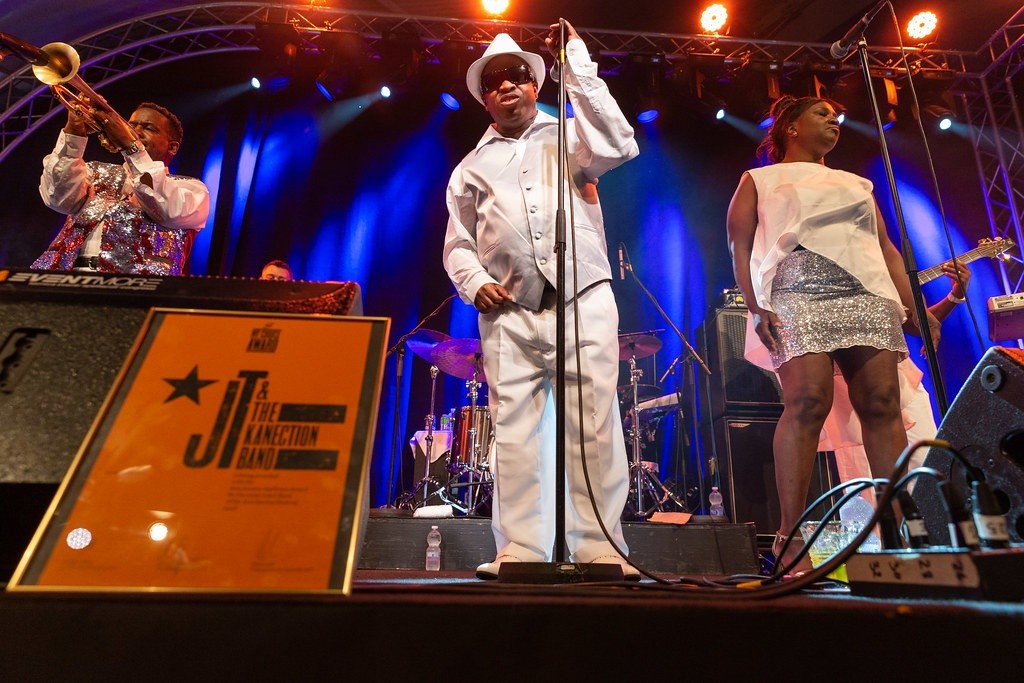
[443,17,641,580]
[727,94,972,594]
[262,260,293,281]
[29,90,210,277]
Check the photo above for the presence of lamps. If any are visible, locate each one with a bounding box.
[622,51,957,132]
[251,30,485,111]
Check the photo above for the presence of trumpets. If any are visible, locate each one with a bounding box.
[32,43,139,153]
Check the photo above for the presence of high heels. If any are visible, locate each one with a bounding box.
[772,531,813,577]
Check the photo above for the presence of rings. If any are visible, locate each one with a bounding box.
[958,271,960,273]
[102,119,108,124]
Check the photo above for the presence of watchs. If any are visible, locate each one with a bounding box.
[125,140,145,156]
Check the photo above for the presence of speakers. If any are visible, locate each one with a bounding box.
[696,307,839,536]
[902,342,1024,544]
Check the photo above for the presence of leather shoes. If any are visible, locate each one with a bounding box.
[475,555,522,580]
[590,556,641,581]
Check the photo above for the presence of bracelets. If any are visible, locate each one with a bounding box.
[947,292,965,304]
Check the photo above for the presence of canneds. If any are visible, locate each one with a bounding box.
[441,416,448,430]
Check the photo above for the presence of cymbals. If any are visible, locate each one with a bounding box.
[432,338,486,381]
[406,329,452,366]
[617,333,663,361]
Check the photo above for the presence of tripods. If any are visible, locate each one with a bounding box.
[397,356,493,517]
[618,345,686,523]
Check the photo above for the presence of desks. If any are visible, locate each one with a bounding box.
[408,430,449,504]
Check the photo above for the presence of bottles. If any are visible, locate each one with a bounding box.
[425,526,442,571]
[838,485,882,553]
[440,408,456,430]
[709,487,724,515]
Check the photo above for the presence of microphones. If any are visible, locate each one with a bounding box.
[619,246,625,280]
[831,0,887,60]
[659,357,680,383]
[551,21,568,83]
[0,32,50,66]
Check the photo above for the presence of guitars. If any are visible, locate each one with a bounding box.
[916,235,1014,283]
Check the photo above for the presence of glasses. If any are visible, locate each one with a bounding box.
[480,65,536,106]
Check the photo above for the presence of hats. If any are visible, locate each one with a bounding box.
[466,33,545,107]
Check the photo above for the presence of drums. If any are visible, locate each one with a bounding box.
[453,406,492,466]
[619,384,661,453]
